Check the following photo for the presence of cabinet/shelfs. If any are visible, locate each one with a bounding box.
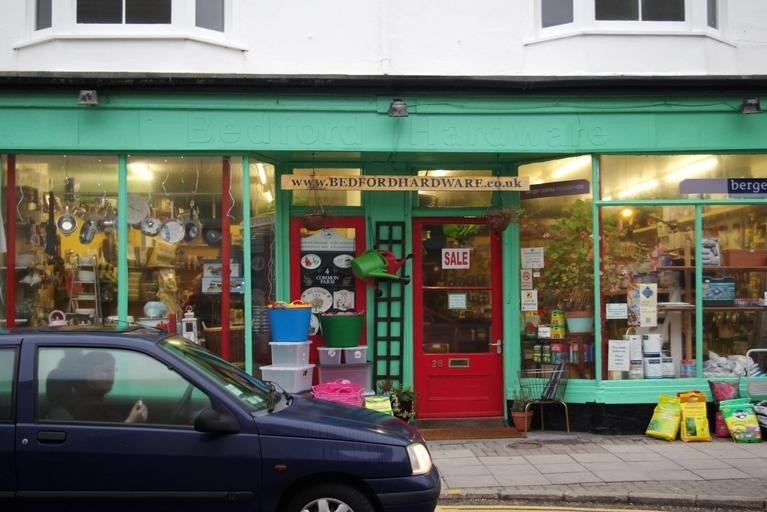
[515,194,766,379]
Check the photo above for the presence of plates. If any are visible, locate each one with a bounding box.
[307,314,320,335]
[301,254,322,269]
[300,287,333,313]
[333,254,354,269]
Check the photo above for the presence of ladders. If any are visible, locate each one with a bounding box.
[68,255,103,317]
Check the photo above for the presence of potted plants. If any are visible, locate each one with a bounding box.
[375,376,419,425]
[508,386,535,432]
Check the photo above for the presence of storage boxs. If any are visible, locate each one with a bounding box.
[254,301,376,409]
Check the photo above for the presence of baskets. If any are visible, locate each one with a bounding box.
[516,369,570,403]
[202,322,244,361]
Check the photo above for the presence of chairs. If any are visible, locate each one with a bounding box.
[512,353,571,441]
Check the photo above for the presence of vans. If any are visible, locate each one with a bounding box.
[422,302,489,355]
[0,330,441,511]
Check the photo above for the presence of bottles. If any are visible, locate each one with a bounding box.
[542,346,550,361]
[532,345,541,362]
[582,343,589,362]
[572,342,579,363]
[168,312,176,332]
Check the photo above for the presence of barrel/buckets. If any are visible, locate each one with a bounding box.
[266,300,312,342]
[565,310,594,334]
[318,309,366,349]
[624,326,675,379]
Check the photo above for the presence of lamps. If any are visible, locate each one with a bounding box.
[387,94,410,118]
[77,85,99,110]
[733,94,764,116]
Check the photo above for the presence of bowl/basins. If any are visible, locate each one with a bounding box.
[720,248,766,266]
[565,311,594,334]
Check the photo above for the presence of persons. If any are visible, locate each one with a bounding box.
[44,365,149,427]
[74,353,131,424]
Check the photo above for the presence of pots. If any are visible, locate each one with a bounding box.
[57,202,197,245]
[200,198,222,246]
